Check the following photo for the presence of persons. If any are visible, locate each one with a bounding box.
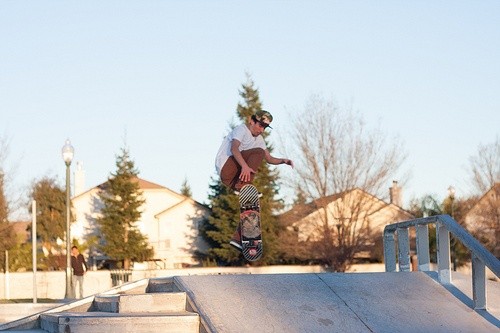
[214,109,295,251]
[71,246,88,300]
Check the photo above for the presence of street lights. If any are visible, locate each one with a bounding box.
[61,138,77,301]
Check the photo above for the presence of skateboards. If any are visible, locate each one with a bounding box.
[239,184,263,261]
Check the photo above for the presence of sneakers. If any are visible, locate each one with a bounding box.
[233,188,264,198]
[229,239,243,250]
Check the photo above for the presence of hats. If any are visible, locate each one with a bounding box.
[254,110,272,130]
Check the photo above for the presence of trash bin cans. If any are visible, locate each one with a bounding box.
[110,271,132,287]
